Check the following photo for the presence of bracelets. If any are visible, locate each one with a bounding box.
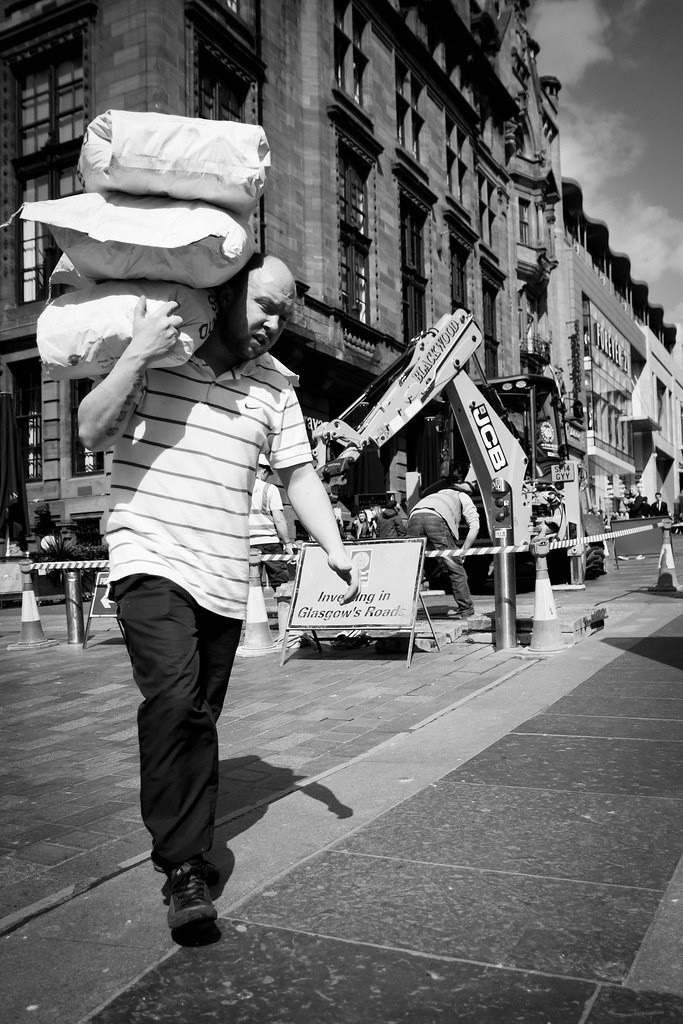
[284,542,292,546]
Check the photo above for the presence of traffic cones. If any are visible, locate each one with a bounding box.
[648,528,682,593]
[6,564,59,652]
[236,551,282,658]
[528,555,571,659]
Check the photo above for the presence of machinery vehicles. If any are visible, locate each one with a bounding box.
[310,307,606,587]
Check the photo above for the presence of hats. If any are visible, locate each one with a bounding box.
[258,454,274,476]
[449,482,474,496]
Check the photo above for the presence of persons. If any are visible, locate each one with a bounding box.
[642,496,651,518]
[291,500,407,569]
[650,492,669,517]
[622,484,643,519]
[243,452,294,596]
[618,489,634,519]
[404,482,480,621]
[668,513,683,535]
[34,520,57,554]
[585,507,630,534]
[76,249,364,934]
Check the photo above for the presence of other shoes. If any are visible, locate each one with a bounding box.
[151,848,219,886]
[161,862,217,929]
